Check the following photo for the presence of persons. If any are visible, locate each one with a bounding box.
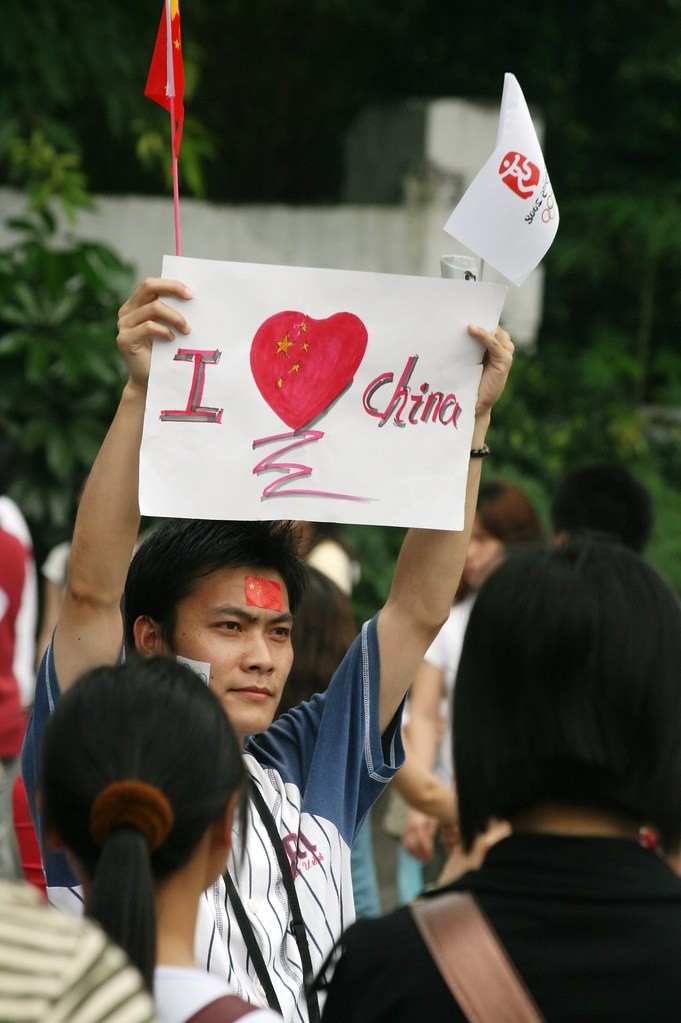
[0,276,681,1023]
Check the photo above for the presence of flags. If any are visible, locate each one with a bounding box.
[442,72,560,287]
[144,0,185,181]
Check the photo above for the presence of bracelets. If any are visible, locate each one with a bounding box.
[470,443,490,457]
